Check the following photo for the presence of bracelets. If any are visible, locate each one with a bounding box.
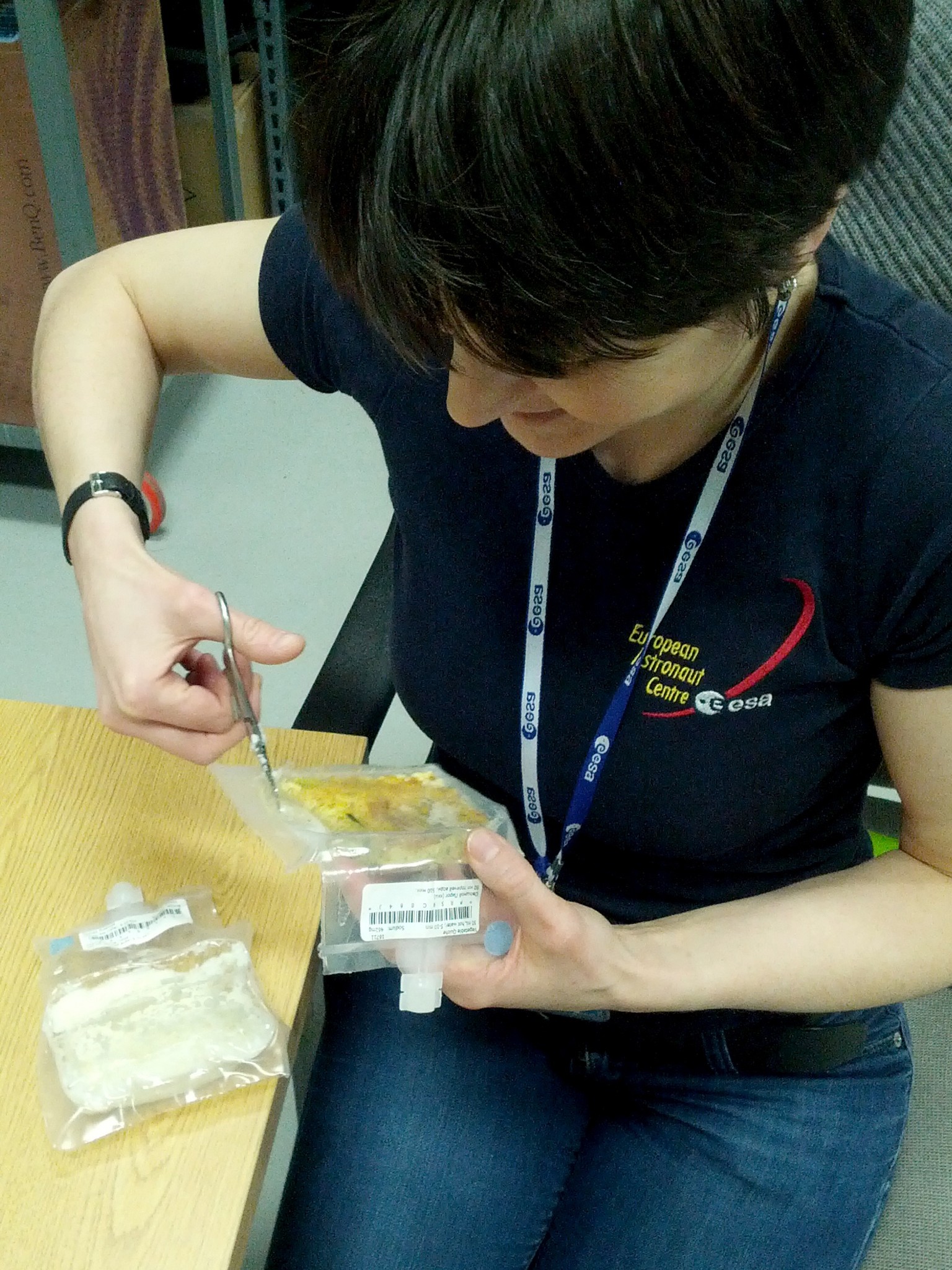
[61,471,150,567]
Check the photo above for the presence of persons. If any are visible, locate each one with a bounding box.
[30,0,951,1270]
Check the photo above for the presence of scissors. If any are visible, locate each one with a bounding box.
[216,591,281,813]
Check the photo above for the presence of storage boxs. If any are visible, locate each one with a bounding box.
[171,49,266,228]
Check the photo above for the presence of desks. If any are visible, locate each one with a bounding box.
[0,700,368,1270]
